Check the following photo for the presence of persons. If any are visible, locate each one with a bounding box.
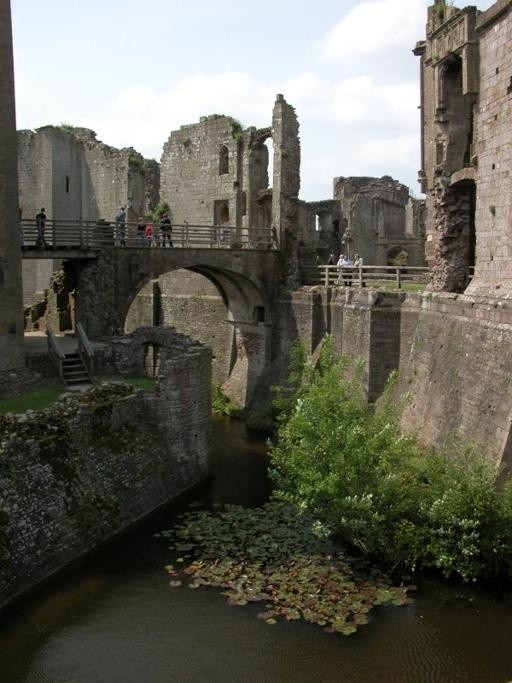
[144,224,154,246]
[18,204,24,246]
[314,252,366,287]
[36,207,46,250]
[115,206,126,247]
[160,214,174,247]
[137,217,145,245]
